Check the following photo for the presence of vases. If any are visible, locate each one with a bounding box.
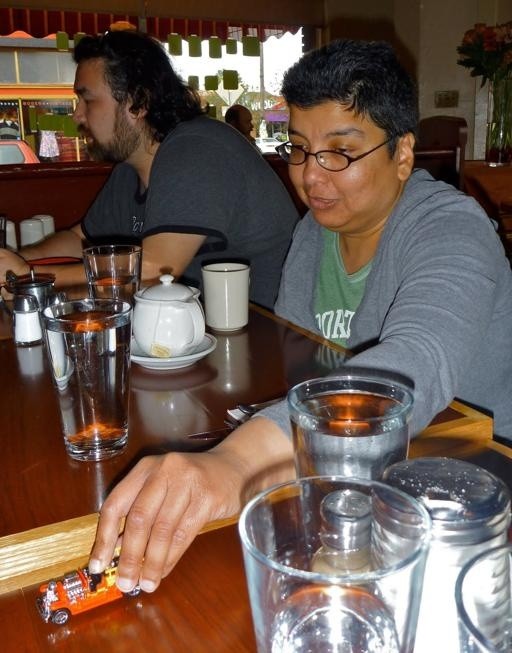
[486,79,512,167]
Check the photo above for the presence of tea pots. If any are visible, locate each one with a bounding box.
[131,273,206,358]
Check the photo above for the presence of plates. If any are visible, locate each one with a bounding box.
[130,333,217,371]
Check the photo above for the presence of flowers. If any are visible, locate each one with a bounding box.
[458,19,512,148]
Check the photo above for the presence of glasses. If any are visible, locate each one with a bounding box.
[275,137,396,172]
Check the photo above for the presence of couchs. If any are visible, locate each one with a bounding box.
[459,158,512,255]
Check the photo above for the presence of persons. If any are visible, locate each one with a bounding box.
[225,105,261,155]
[82,37,512,593]
[0,30,301,312]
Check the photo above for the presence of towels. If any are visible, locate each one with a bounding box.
[228,396,286,423]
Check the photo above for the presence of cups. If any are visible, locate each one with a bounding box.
[32,215,55,237]
[40,298,133,463]
[454,543,511,652]
[200,263,252,332]
[81,245,141,302]
[6,220,18,253]
[236,474,432,653]
[19,218,45,248]
[287,375,415,567]
